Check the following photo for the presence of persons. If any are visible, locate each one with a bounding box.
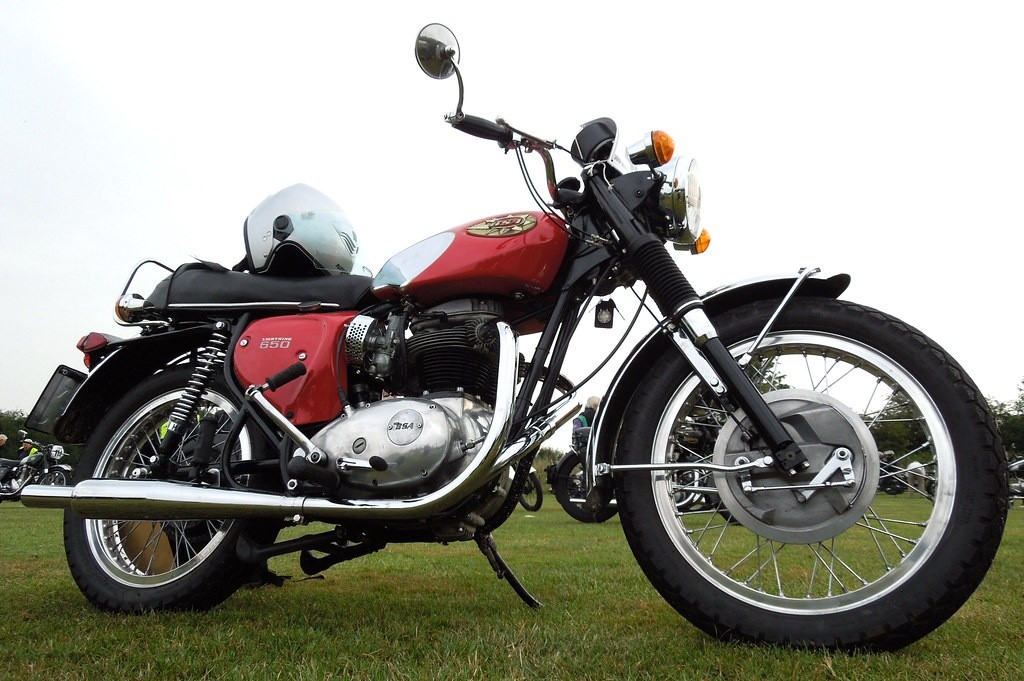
[0,434,8,446]
[905,461,926,498]
[16,439,38,461]
[882,450,897,490]
[572,396,602,446]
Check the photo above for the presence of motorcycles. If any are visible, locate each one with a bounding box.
[518,410,1024,524]
[20,23,1011,656]
[0,431,74,504]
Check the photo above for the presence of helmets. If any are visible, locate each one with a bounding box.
[584,396,601,411]
[884,450,895,459]
[242,181,360,276]
